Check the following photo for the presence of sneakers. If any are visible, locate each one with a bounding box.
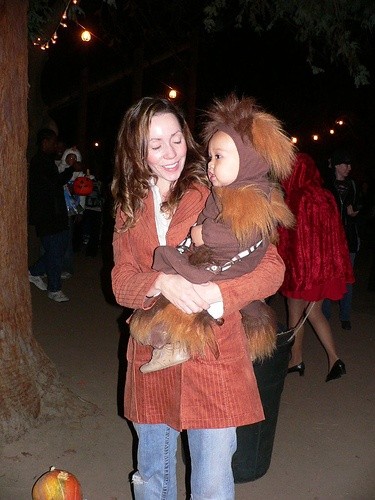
[140,341,191,373]
[47,289,70,302]
[27,269,48,290]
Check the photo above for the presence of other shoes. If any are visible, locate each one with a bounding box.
[341,321,351,328]
[45,271,72,279]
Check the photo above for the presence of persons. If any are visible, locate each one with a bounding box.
[263,148,375,382]
[110,97,286,500]
[136,96,296,375]
[29,109,119,302]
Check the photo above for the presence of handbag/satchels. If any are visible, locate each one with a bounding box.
[74,177,94,195]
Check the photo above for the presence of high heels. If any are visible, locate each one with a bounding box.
[287,362,304,376]
[325,358,346,382]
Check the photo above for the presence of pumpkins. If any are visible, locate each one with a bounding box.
[32,467,82,500]
